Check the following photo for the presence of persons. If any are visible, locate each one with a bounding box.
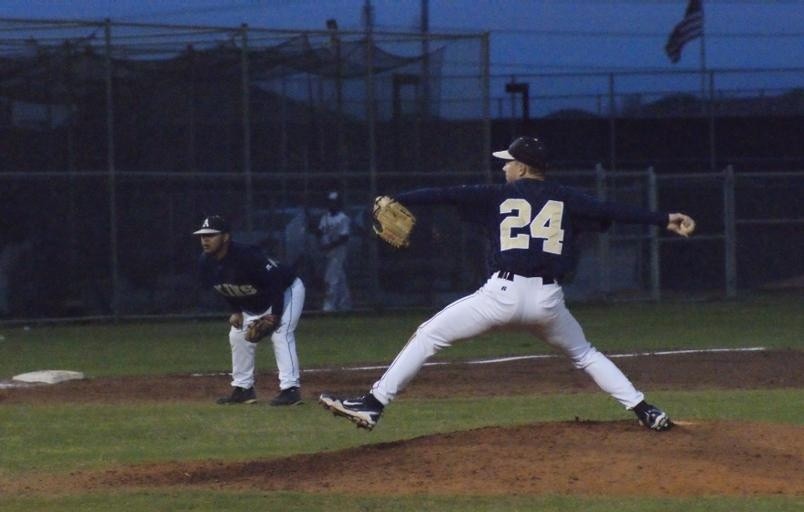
[188,214,307,407]
[319,135,695,433]
[316,188,355,313]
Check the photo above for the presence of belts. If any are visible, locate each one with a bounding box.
[498,270,554,285]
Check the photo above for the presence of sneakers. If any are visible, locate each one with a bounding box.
[318,393,381,431]
[217,387,257,404]
[638,405,671,431]
[271,389,304,406]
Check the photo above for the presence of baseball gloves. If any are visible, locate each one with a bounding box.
[372,196,417,247]
[244,314,286,343]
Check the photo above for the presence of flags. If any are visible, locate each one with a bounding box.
[665,0,707,64]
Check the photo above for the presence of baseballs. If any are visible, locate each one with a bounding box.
[680,220,695,234]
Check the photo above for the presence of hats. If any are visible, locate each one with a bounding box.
[490,135,549,168]
[325,191,341,204]
[192,215,231,235]
